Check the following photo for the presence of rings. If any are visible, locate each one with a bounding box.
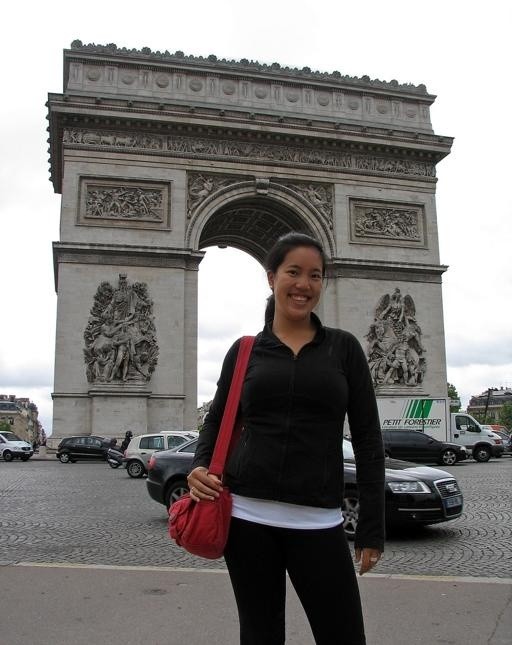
[190,487,197,493]
[370,556,379,565]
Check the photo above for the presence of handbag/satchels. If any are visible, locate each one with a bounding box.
[167,472,233,558]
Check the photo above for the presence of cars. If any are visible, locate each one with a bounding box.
[339,434,465,540]
[146,437,200,514]
[459,425,512,458]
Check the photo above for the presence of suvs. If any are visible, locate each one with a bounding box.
[122,434,191,478]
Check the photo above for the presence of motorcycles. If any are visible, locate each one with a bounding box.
[100,437,126,469]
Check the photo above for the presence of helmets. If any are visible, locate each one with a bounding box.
[125,430,133,438]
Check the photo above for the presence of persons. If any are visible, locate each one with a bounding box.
[292,183,333,228]
[86,186,163,218]
[185,233,387,645]
[187,172,215,218]
[95,273,151,381]
[370,285,426,385]
[356,208,420,238]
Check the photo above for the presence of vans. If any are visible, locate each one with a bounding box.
[157,429,199,449]
[380,426,470,466]
[56,435,122,463]
[0,430,34,461]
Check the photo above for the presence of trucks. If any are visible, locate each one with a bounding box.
[376,395,504,463]
